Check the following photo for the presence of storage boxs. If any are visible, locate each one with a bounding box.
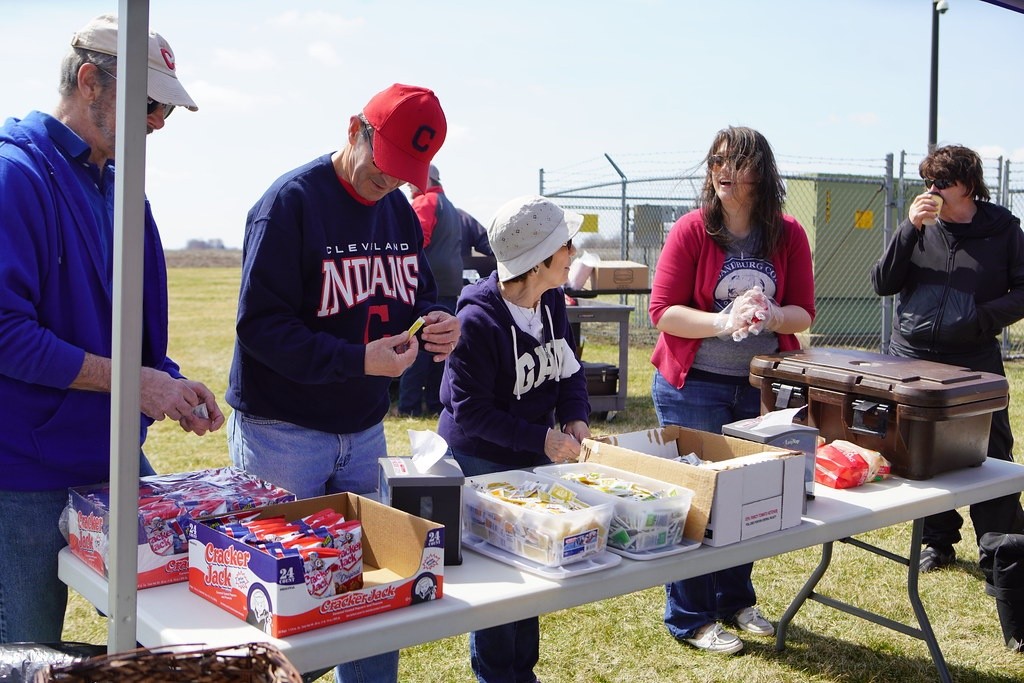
[67,465,300,594]
[576,423,808,548]
[581,362,619,395]
[590,261,649,290]
[187,488,447,642]
[462,468,617,567]
[530,461,696,550]
[747,346,1012,478]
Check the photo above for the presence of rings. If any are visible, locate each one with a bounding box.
[449,342,455,350]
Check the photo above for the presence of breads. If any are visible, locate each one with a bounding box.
[817,436,891,482]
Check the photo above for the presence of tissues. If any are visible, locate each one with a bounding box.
[721,402,820,502]
[377,427,467,564]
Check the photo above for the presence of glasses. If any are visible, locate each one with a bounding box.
[706,154,752,173]
[561,239,572,252]
[95,65,175,121]
[361,123,384,172]
[924,178,960,190]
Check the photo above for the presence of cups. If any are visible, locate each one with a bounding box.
[920,192,944,226]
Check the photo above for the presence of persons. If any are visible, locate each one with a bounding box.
[389,166,497,419]
[1,14,225,659]
[647,125,816,657]
[226,83,462,683]
[438,196,592,683]
[871,140,1024,597]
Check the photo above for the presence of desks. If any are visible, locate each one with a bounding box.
[566,298,636,422]
[563,288,652,344]
[58,454,1024,683]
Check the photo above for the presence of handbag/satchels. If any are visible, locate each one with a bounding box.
[979,531,1024,653]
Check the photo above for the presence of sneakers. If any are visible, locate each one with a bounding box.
[918,545,956,572]
[682,621,744,653]
[734,605,774,636]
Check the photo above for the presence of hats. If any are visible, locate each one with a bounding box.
[487,193,585,281]
[70,14,200,114]
[363,84,448,197]
[427,163,440,187]
